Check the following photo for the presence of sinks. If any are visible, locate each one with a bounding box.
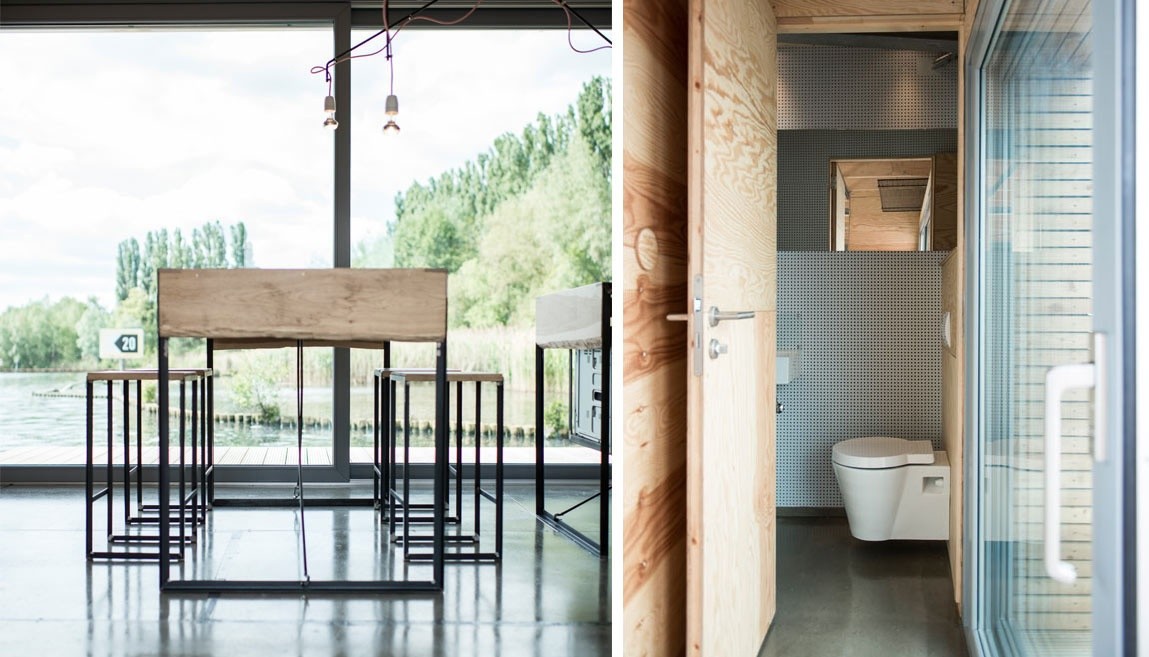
[776,350,801,385]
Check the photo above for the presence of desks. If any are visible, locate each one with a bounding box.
[157,266,449,595]
[536,283,612,559]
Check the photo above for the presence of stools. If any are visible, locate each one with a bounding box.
[373,368,504,563]
[85,368,216,560]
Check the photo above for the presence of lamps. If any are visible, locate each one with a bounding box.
[378,0,401,136]
[311,64,338,130]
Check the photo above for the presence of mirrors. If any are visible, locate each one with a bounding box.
[777,128,957,252]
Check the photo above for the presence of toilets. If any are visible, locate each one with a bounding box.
[830,435,951,541]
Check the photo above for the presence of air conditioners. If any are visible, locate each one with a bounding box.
[573,347,605,441]
